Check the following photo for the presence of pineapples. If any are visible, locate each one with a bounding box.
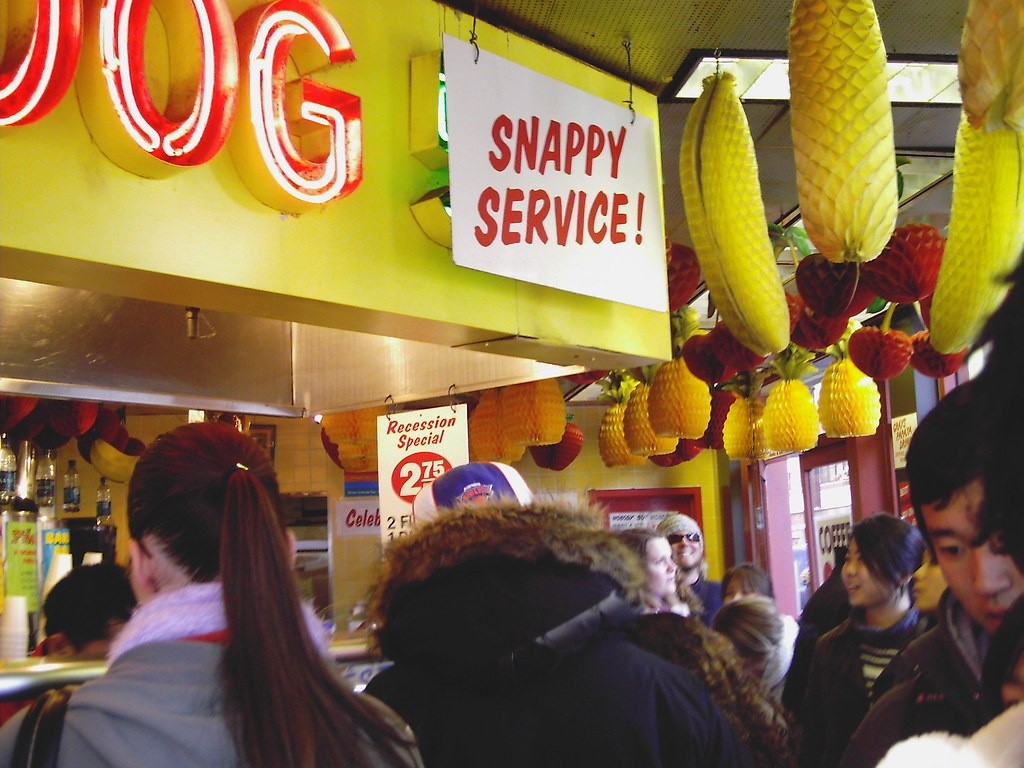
[324,307,881,470]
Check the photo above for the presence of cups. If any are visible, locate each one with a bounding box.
[0,595,28,661]
[38,549,73,646]
[80,552,103,566]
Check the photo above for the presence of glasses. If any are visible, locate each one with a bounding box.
[666,532,700,544]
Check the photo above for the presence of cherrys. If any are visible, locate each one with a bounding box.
[650,222,965,469]
[0,396,147,462]
[529,414,583,470]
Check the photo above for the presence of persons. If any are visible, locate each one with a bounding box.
[791,510,958,768]
[712,565,801,705]
[842,247,1024,768]
[0,422,426,768]
[354,459,740,767]
[26,562,137,659]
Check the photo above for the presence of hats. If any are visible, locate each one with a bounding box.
[655,512,703,551]
[411,462,538,533]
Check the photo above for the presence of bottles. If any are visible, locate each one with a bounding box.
[62,459,80,512]
[37,448,55,520]
[96,477,112,526]
[0,432,16,503]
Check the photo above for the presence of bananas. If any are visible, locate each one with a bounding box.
[89,440,142,483]
[676,0,1023,359]
